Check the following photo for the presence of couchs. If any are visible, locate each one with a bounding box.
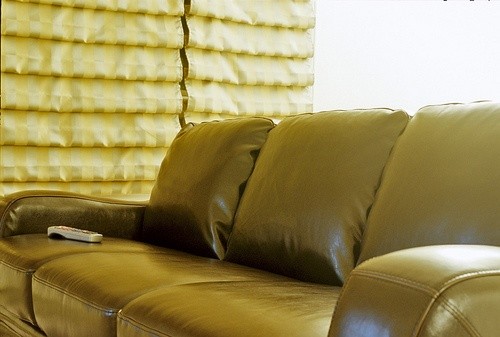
[0,100,500,336]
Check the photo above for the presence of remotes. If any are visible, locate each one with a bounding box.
[47,226,103,242]
